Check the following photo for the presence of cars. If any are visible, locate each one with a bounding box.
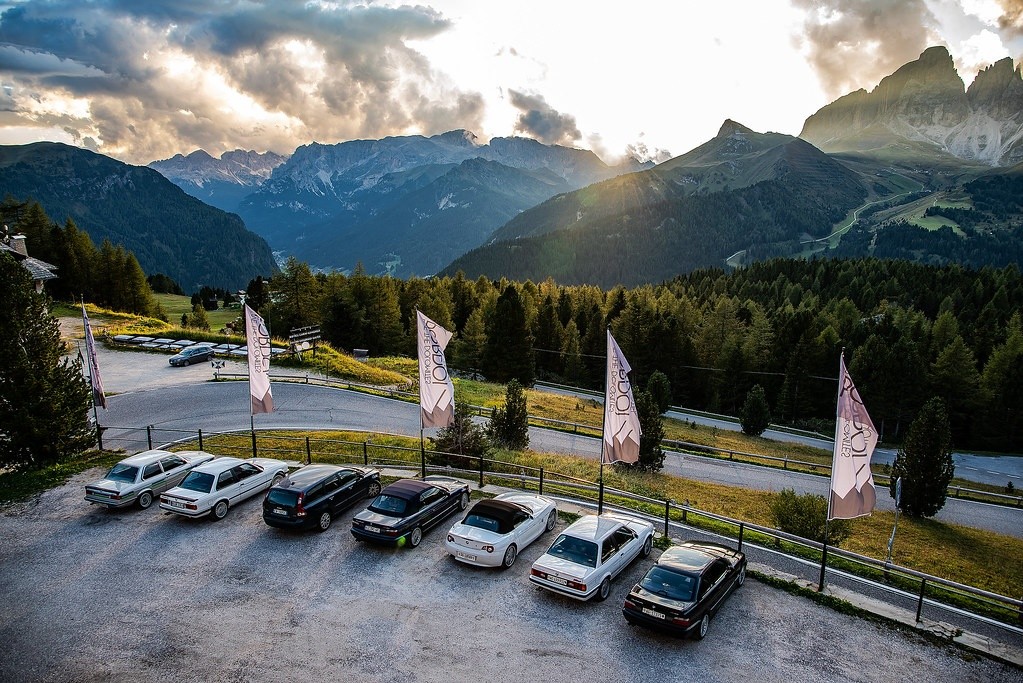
[621,540,747,642]
[529,511,656,603]
[349,475,471,549]
[168,345,214,367]
[445,492,557,571]
[158,457,290,522]
[83,450,215,510]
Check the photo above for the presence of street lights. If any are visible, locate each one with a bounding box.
[262,280,273,357]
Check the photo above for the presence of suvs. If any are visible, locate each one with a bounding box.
[262,463,381,534]
